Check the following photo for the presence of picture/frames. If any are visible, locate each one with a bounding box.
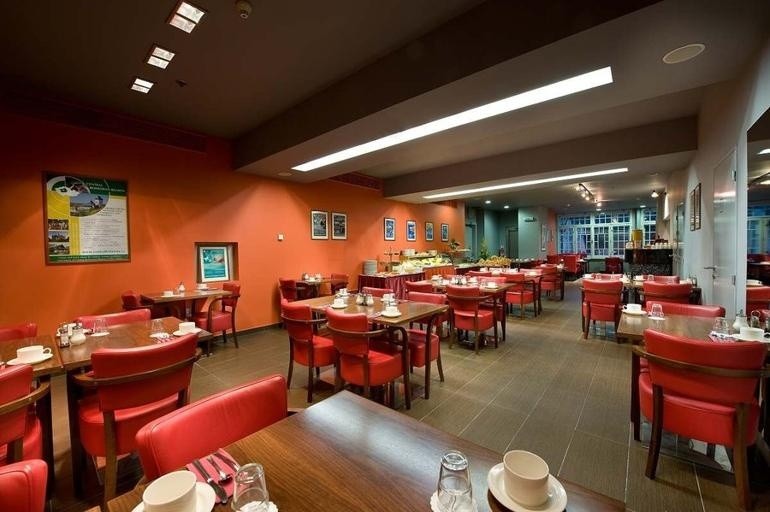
[425,221,434,241]
[689,189,695,230]
[441,224,449,242]
[694,183,701,230]
[331,212,348,240]
[406,220,416,242]
[42,170,130,265]
[198,245,230,283]
[384,218,396,241]
[310,210,329,240]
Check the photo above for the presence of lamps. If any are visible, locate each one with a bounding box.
[651,190,659,198]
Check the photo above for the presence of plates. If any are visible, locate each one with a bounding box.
[582,277,594,279]
[363,260,377,275]
[428,250,437,256]
[621,309,647,315]
[380,299,395,302]
[402,249,415,256]
[485,286,499,289]
[632,279,647,282]
[6,354,53,366]
[56,328,90,337]
[381,311,402,318]
[732,333,770,343]
[330,305,348,309]
[172,328,202,337]
[161,295,181,298]
[746,284,763,286]
[131,482,216,512]
[487,462,568,512]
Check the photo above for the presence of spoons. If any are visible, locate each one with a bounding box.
[205,456,233,486]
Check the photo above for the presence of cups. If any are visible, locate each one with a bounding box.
[648,275,655,281]
[141,469,198,512]
[230,462,270,512]
[151,319,165,337]
[334,299,344,306]
[622,303,641,313]
[746,280,762,284]
[481,280,486,288]
[383,294,390,300]
[651,304,664,318]
[197,284,206,289]
[502,450,550,507]
[437,450,474,512]
[713,317,728,335]
[487,282,498,287]
[636,276,643,279]
[739,327,770,341]
[68,322,82,335]
[692,275,697,288]
[179,322,196,334]
[93,318,106,333]
[596,274,601,280]
[339,288,347,294]
[163,291,174,296]
[386,307,397,315]
[585,274,591,278]
[16,345,51,363]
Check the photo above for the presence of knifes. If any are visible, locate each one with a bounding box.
[192,460,229,504]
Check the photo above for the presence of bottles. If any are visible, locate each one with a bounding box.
[355,292,374,306]
[59,322,70,348]
[655,243,672,249]
[70,320,86,345]
[454,275,466,284]
[732,309,749,332]
[179,281,185,292]
[383,300,390,310]
